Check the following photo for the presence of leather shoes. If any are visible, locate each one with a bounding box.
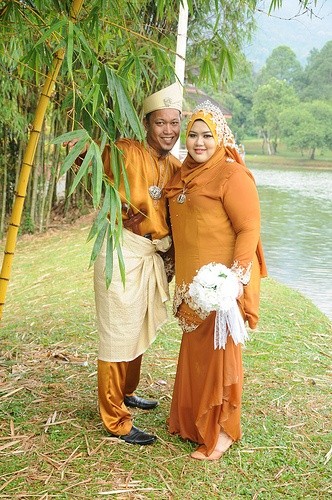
[124,394,160,410]
[110,424,157,445]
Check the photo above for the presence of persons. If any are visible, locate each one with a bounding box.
[164,99,268,460]
[62,81,185,444]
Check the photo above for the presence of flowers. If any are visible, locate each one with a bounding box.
[190,261,252,349]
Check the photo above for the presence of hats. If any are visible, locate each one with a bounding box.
[143,80,183,115]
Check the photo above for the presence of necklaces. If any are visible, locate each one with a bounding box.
[176,187,190,204]
[142,142,172,199]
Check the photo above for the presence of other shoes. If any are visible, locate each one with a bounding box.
[193,434,236,462]
